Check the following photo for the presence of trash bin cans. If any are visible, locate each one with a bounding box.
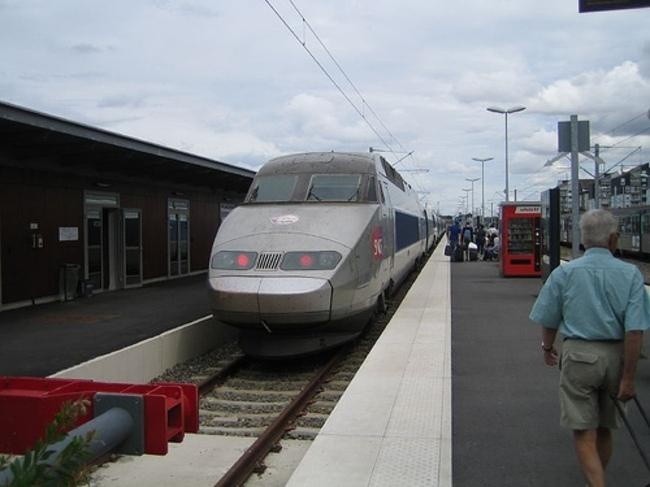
[58,263,80,301]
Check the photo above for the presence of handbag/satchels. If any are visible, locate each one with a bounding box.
[444,244,453,256]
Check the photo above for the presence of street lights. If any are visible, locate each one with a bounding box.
[486,103,526,201]
[472,156,494,224]
[454,187,473,217]
[465,176,481,217]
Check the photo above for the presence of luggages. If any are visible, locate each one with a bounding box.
[466,249,478,261]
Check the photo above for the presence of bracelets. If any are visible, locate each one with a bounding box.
[541,339,554,351]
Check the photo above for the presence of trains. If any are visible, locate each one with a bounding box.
[560,204,650,258]
[208,149,449,371]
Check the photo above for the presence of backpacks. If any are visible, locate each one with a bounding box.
[464,229,472,242]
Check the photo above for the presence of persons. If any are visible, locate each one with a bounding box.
[528,208,650,486]
[445,214,501,261]
